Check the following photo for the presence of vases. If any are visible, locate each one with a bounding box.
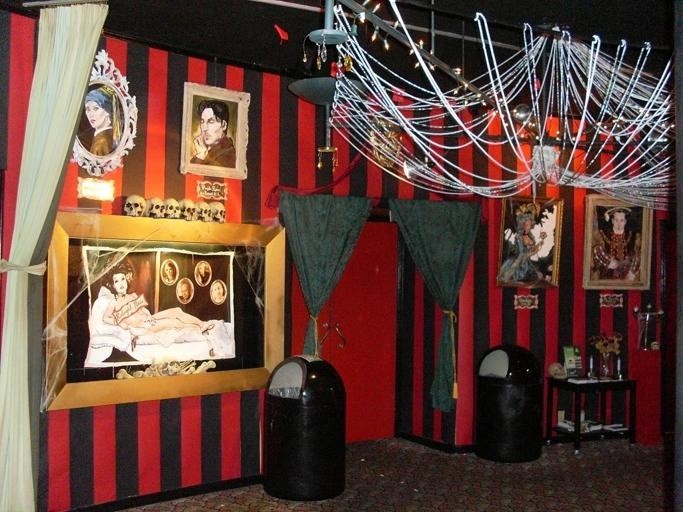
[599,353,612,380]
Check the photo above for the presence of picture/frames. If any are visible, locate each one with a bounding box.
[179,83,250,180]
[70,49,139,177]
[46,213,286,411]
[496,196,565,289]
[581,194,652,291]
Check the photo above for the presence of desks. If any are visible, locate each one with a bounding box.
[546,376,636,455]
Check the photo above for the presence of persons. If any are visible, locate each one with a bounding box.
[503,215,545,285]
[84,86,125,155]
[192,98,238,168]
[87,261,234,363]
[592,206,641,280]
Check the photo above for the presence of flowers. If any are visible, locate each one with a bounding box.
[587,332,623,355]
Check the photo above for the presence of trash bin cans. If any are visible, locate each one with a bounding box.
[474,343,543,463]
[263,353,347,502]
